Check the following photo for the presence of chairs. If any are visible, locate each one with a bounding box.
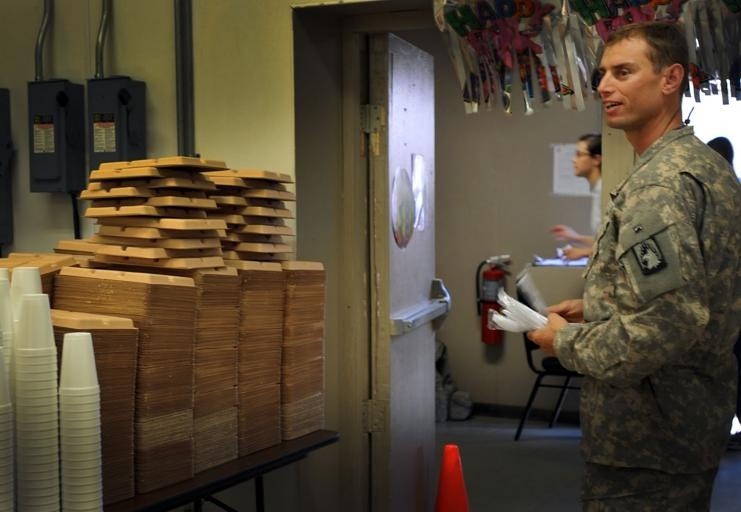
[515,270,585,441]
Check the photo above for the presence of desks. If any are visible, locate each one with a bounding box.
[101,428,342,512]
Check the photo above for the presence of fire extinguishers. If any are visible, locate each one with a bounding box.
[476,255,512,343]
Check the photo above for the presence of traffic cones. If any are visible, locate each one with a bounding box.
[436,443,469,512]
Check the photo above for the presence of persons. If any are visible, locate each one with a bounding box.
[547,133,603,266]
[706,135,741,452]
[522,16,741,512]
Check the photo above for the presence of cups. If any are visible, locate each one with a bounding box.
[0,264,105,512]
